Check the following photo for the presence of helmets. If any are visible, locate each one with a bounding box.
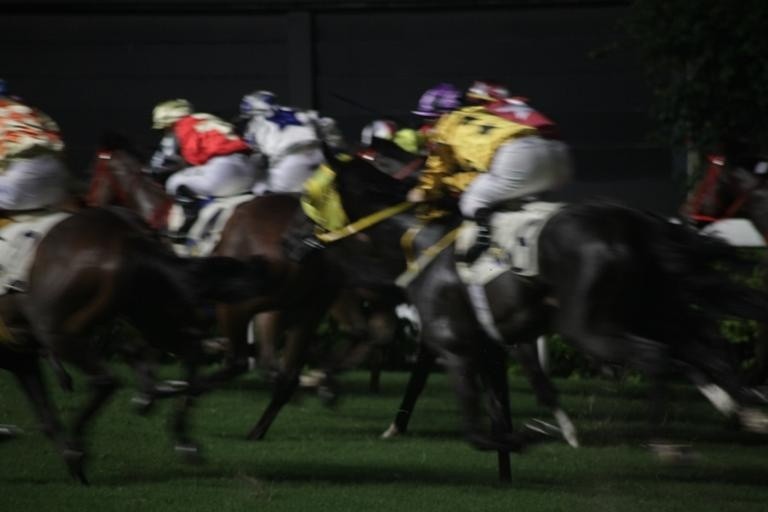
[360,120,432,152]
[466,79,509,105]
[412,85,464,120]
[151,96,193,131]
[238,91,282,121]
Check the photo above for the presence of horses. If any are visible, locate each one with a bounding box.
[1,127,768,488]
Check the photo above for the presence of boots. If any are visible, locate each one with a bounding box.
[461,206,494,264]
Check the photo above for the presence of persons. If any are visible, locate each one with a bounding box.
[0,77,81,211]
[335,119,460,211]
[150,96,267,240]
[238,91,345,196]
[405,81,573,268]
